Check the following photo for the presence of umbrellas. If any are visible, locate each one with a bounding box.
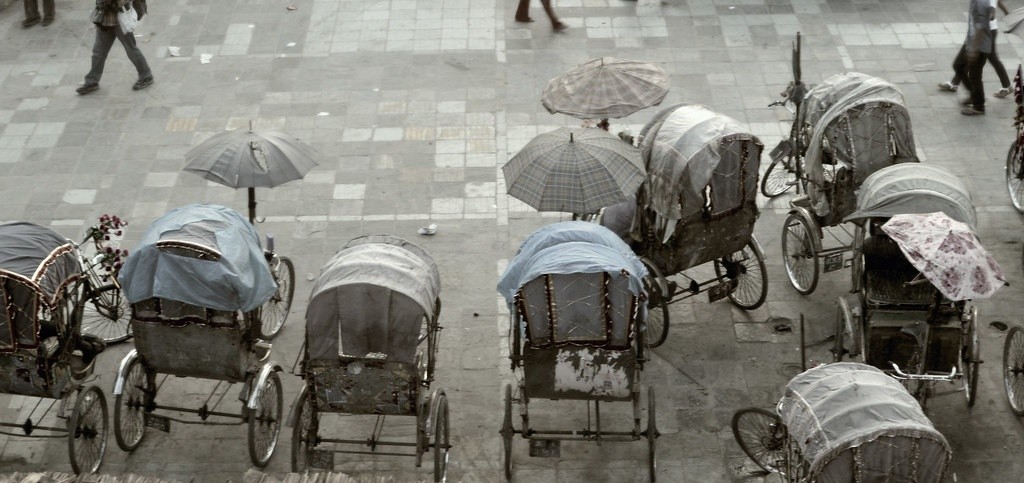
[182,120,320,225]
[501,126,648,215]
[880,211,1008,301]
[541,56,672,119]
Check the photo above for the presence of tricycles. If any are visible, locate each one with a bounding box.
[1004,64,1024,211]
[113,203,296,469]
[829,162,988,406]
[496,221,664,483]
[758,70,920,294]
[730,363,953,483]
[594,104,770,349]
[278,233,453,483]
[1,218,136,478]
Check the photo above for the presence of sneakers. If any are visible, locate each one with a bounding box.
[994,86,1013,97]
[76,83,99,94]
[22,17,40,27]
[42,18,53,25]
[938,81,958,92]
[133,75,153,90]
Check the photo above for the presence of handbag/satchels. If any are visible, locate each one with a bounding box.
[132,0,147,21]
[117,6,138,35]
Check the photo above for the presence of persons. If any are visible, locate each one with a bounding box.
[75,0,155,95]
[21,0,55,28]
[515,0,569,32]
[937,0,1015,115]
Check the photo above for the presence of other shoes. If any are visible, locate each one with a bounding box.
[960,99,985,115]
[517,18,534,22]
[554,25,567,30]
